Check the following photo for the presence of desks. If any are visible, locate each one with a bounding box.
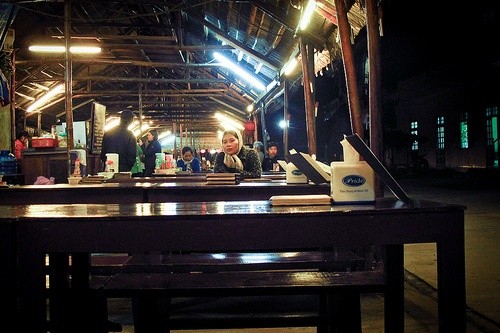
[21,147,71,185]
[0,170,467,333]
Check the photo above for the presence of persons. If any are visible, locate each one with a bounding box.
[244,141,285,172]
[15,131,30,160]
[100,111,161,177]
[214,129,261,179]
[177,147,200,173]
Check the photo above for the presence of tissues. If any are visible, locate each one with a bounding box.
[285,149,309,184]
[330,133,375,201]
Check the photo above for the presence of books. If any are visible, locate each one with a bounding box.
[81,176,104,184]
[206,173,240,185]
[269,195,331,206]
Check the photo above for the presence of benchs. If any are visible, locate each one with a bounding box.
[45,251,385,333]
